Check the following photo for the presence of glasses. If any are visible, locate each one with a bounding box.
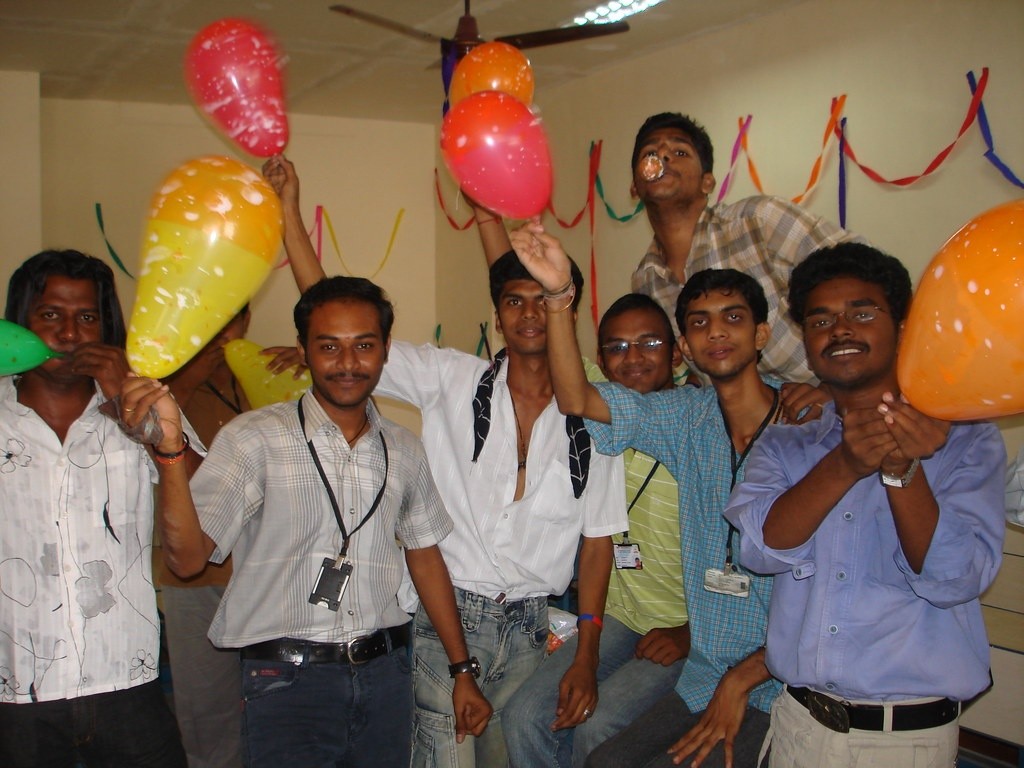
[600,339,670,357]
[804,304,895,332]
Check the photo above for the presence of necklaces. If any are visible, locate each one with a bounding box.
[509,391,528,471]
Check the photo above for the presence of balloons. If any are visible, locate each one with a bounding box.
[440,40,553,221]
[0,318,64,377]
[224,339,312,410]
[184,19,290,159]
[124,157,286,379]
[896,200,1024,422]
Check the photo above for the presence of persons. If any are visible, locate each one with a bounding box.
[263,155,629,768]
[635,557,640,569]
[459,186,1007,768]
[117,275,495,768]
[740,582,746,590]
[629,112,871,426]
[0,248,254,768]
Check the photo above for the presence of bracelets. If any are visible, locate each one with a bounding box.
[542,280,576,313]
[152,431,189,465]
[577,615,603,630]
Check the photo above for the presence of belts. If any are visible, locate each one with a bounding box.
[786,685,959,734]
[240,617,413,665]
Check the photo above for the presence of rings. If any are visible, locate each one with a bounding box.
[123,408,135,413]
[815,402,824,407]
[584,710,591,716]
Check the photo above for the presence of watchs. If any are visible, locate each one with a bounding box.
[880,457,920,488]
[448,656,481,680]
